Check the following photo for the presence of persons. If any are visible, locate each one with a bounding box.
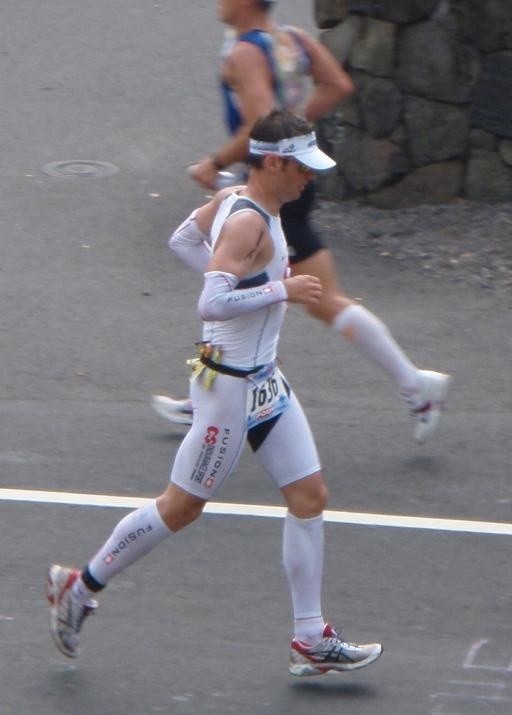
[145,0,455,447]
[42,110,385,679]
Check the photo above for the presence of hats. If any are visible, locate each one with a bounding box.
[248,131,336,170]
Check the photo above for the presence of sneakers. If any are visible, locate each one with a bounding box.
[47,565,97,657]
[291,623,380,676]
[154,395,194,425]
[400,370,447,445]
